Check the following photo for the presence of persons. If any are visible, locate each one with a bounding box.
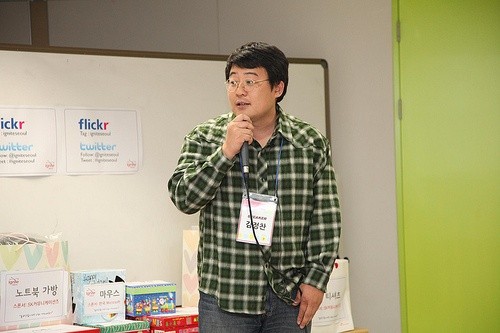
[170,41,342,333]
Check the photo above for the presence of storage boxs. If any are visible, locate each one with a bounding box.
[311,258,355,333]
[0,229,200,333]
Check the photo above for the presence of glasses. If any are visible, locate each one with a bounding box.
[225,78,269,91]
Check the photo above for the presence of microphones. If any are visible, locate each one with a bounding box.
[240,141,250,183]
